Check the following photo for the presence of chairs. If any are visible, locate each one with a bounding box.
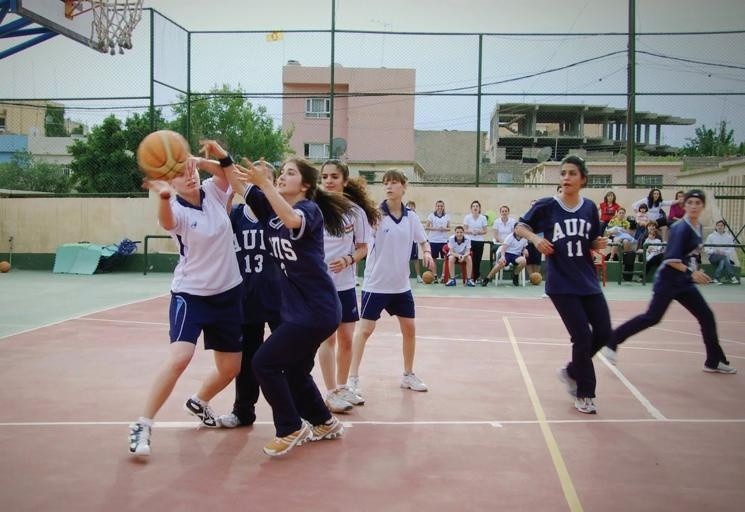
[591,249,606,287]
[443,245,473,285]
[618,250,643,285]
[495,246,525,287]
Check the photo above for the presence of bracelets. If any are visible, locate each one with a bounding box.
[159,192,171,200]
[342,256,348,267]
[347,254,355,265]
[217,152,234,168]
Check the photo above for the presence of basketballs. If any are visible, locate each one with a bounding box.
[530,272,543,285]
[423,271,434,285]
[0,262,8,271]
[136,130,189,181]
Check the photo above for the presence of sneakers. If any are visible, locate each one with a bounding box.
[559,367,597,413]
[184,398,242,428]
[703,362,736,374]
[467,278,488,286]
[128,423,151,457]
[417,276,456,286]
[512,272,518,286]
[401,374,426,391]
[712,276,740,284]
[326,376,364,412]
[263,415,343,456]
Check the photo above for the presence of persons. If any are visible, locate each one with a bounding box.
[405,200,542,286]
[197,139,345,458]
[316,158,368,414]
[128,152,243,459]
[600,188,687,280]
[218,159,281,434]
[600,189,738,374]
[513,154,611,414]
[353,168,434,398]
[704,221,739,285]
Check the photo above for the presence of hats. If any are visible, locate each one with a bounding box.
[685,190,705,202]
